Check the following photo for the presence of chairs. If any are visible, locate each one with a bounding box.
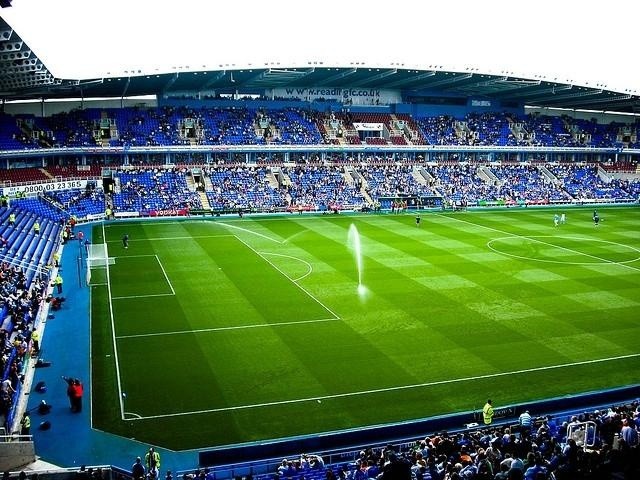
[211,464,354,480]
[1,164,202,224]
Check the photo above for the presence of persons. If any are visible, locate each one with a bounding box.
[1,448,160,480]
[0,211,92,442]
[326,400,640,480]
[0,95,640,229]
[273,453,324,480]
[1,399,31,441]
[121,233,128,249]
[164,469,253,480]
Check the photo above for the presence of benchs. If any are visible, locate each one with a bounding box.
[0,206,60,289]
